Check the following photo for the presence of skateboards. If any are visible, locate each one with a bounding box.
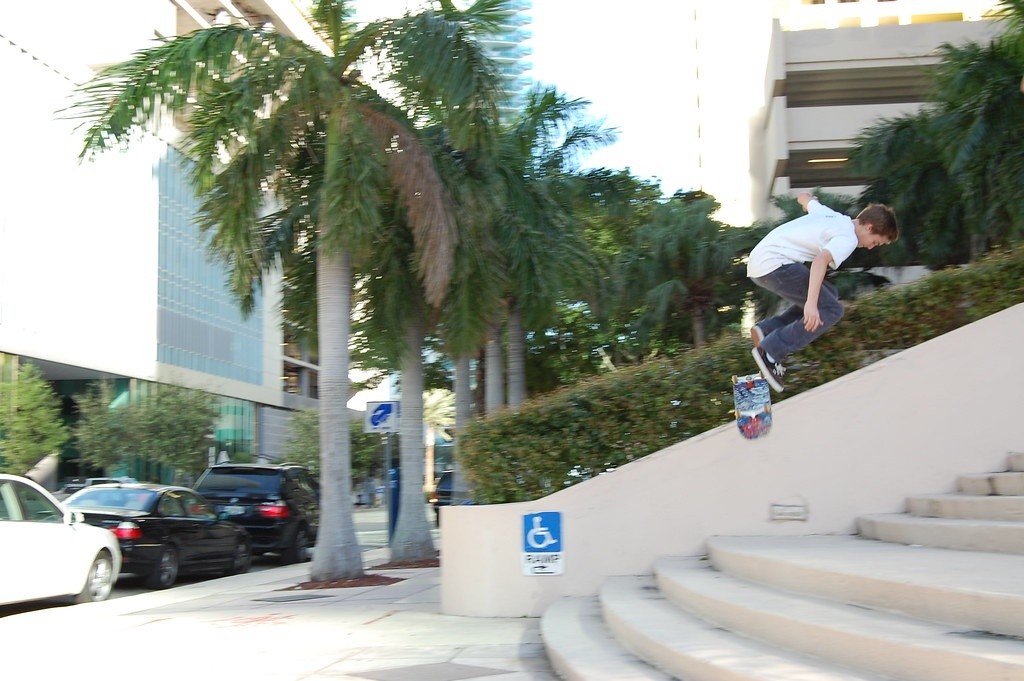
[732,372,774,441]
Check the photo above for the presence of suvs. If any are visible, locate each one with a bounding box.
[191,460,321,565]
[432,470,456,526]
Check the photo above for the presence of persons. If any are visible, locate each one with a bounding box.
[746,192,899,393]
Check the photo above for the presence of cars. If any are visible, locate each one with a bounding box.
[0,472,123,607]
[50,476,139,503]
[59,483,252,591]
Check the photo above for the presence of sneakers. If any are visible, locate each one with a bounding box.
[751,346,787,393]
[750,321,767,347]
